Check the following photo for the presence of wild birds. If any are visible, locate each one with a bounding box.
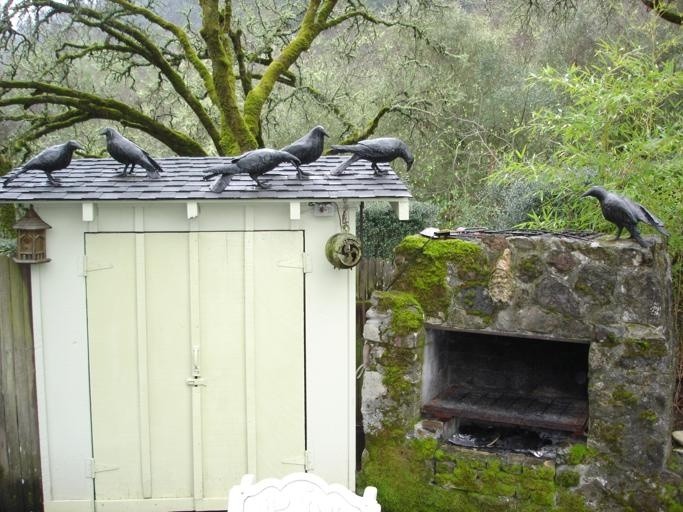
[278,124,331,181]
[8,139,85,187]
[580,186,670,249]
[201,147,302,194]
[98,127,164,178]
[332,137,413,177]
